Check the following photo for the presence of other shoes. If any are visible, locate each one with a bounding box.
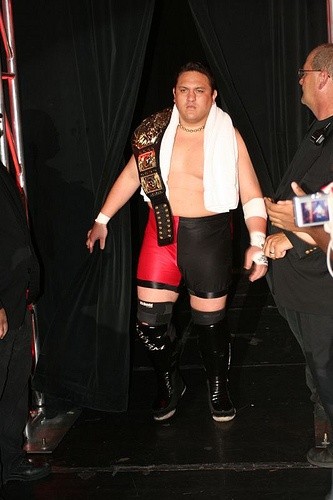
[306,441,333,468]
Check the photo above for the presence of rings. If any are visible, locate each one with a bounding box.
[269,252,275,254]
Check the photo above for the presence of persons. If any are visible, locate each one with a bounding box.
[263,43,333,500]
[0,162,51,487]
[87,59,268,421]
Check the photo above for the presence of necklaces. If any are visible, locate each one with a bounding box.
[179,122,204,132]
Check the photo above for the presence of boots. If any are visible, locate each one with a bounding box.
[136,321,187,420]
[192,322,236,422]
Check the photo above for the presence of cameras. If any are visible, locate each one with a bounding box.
[293,193,333,227]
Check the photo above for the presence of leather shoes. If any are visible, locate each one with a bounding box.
[0,457,51,480]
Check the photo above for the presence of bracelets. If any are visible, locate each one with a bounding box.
[250,232,266,248]
[95,212,110,224]
[242,198,268,222]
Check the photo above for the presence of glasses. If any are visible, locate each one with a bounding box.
[297,68,330,79]
